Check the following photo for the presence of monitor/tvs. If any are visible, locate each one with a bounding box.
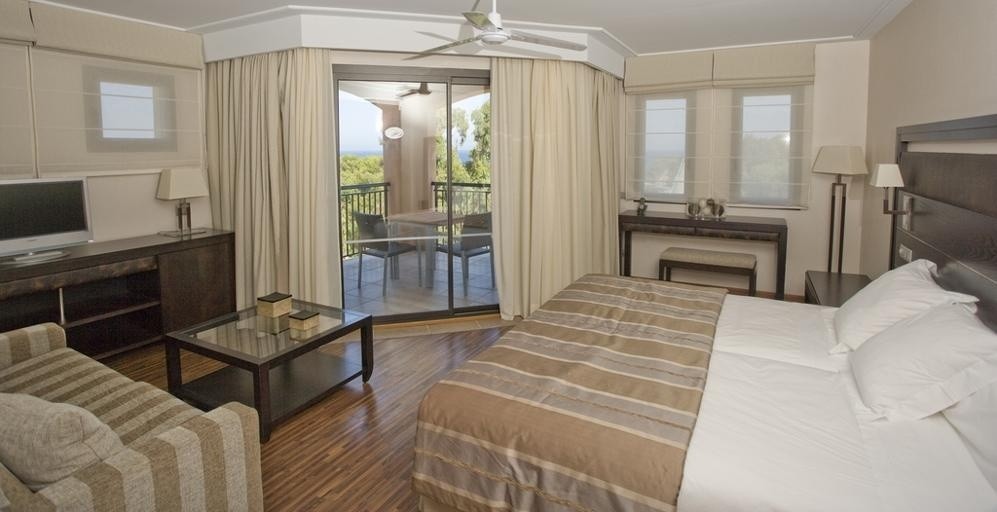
[0,177,94,262]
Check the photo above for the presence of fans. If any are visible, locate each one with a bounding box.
[403,0,588,62]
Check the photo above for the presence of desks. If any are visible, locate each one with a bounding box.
[616,208,789,301]
[378,209,467,291]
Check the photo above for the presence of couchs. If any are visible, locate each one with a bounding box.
[0,319,268,512]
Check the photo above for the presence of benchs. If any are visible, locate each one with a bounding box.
[658,246,758,297]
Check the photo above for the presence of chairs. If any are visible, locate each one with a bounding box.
[433,211,496,297]
[350,208,423,298]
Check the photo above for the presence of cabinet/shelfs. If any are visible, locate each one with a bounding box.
[0,234,238,363]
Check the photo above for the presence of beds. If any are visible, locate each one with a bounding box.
[408,110,997,511]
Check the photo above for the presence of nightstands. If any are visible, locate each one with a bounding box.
[803,269,873,308]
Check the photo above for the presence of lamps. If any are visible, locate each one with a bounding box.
[868,161,916,234]
[153,162,210,240]
[810,142,870,274]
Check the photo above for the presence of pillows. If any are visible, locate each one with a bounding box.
[0,391,126,491]
[820,257,982,356]
[848,299,997,440]
